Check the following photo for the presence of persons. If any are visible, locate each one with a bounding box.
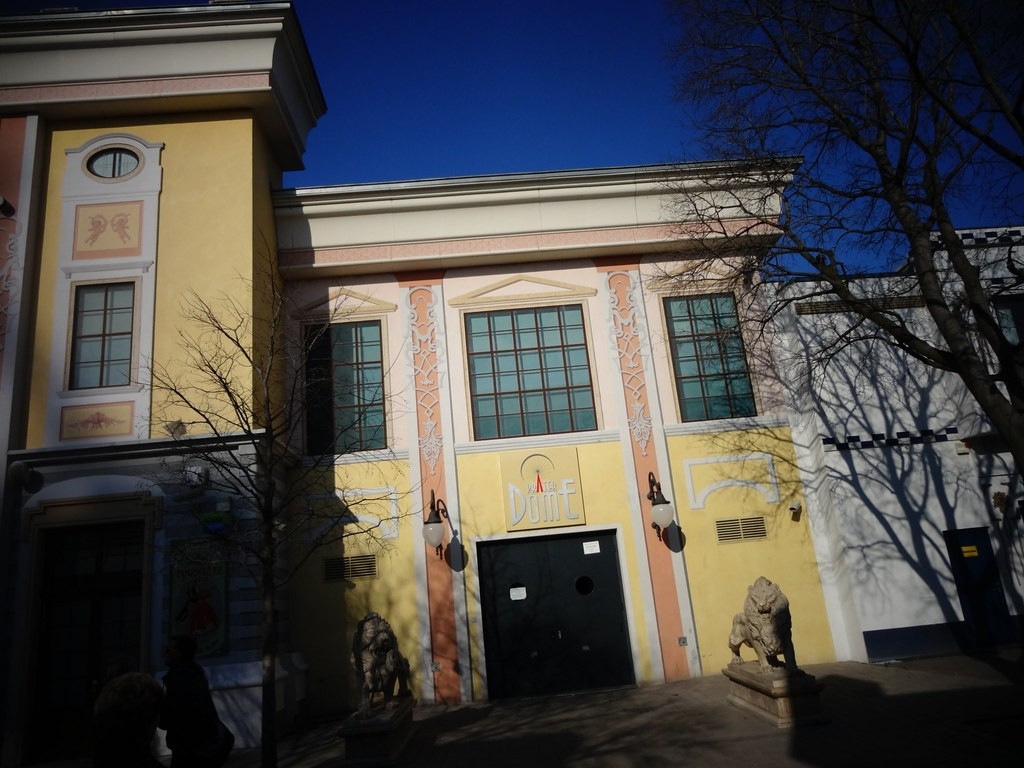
[92,670,168,768]
[151,634,226,768]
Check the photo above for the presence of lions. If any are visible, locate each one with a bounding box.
[729,576,803,675]
[353,612,410,719]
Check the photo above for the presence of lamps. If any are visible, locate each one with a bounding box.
[646,471,674,542]
[422,489,458,561]
